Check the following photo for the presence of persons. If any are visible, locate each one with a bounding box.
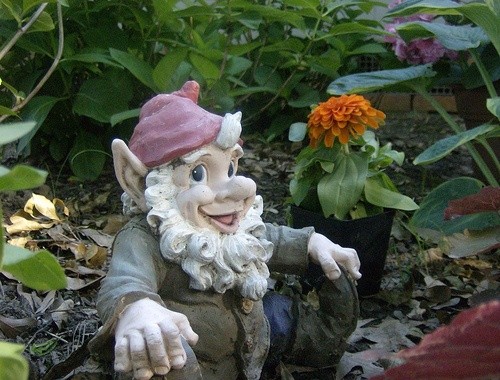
[92,80,362,380]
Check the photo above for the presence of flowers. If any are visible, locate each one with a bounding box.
[283,93,420,221]
[383,0,460,66]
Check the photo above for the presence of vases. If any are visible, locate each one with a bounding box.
[289,206,398,297]
